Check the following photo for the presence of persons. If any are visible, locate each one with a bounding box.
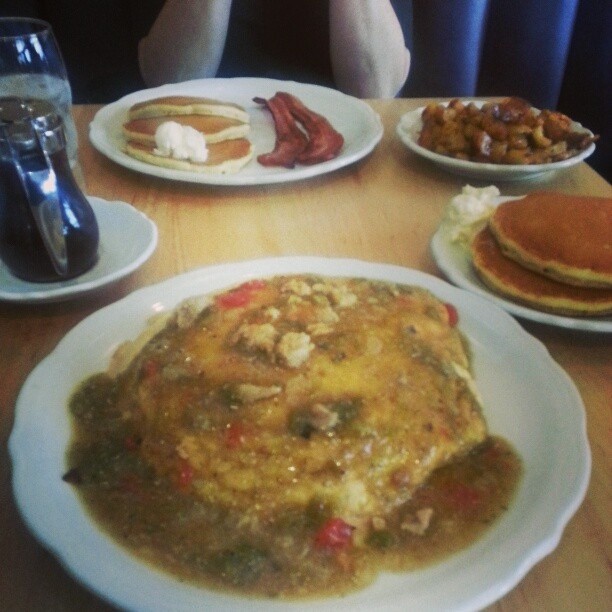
[137,0,411,99]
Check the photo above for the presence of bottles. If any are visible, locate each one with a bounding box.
[0,96,101,284]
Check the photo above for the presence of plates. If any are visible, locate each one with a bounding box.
[431,195,611,333]
[0,193,160,303]
[6,255,594,612]
[396,100,596,182]
[87,76,384,187]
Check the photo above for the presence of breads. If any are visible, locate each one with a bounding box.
[123,137,253,176]
[128,95,249,123]
[121,114,250,147]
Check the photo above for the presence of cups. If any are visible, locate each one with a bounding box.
[0,17,79,170]
